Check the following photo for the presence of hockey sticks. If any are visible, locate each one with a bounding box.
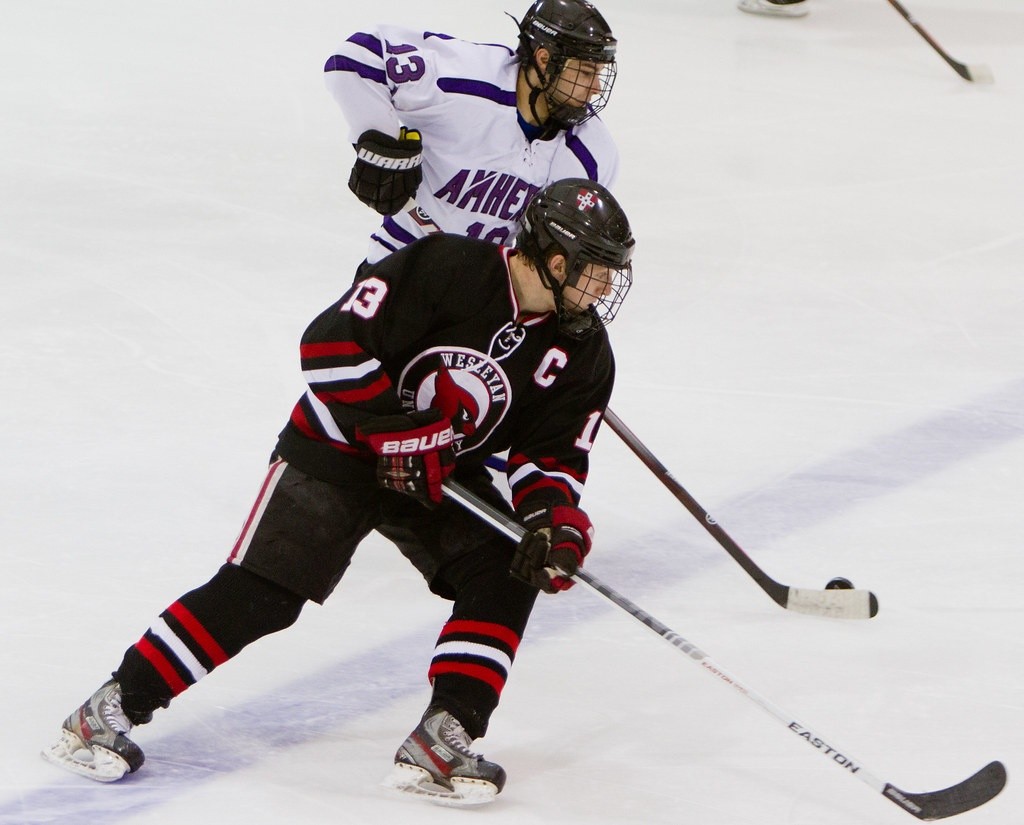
[440,477,1009,823]
[400,198,881,620]
[896,3,1002,86]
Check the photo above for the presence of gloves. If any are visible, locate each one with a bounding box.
[347,129,424,215]
[356,406,456,512]
[509,499,596,594]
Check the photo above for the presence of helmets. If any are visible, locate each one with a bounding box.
[516,177,636,340]
[518,0,618,125]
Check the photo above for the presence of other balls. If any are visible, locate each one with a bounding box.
[822,577,855,589]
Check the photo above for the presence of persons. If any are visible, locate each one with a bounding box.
[41,178,636,806]
[324,0,620,473]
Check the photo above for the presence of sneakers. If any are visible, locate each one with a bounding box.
[384,704,507,806]
[736,0,810,16]
[41,677,145,782]
